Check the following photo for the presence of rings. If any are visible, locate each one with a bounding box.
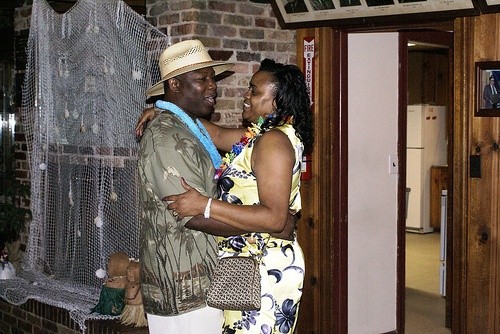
[173,211,178,216]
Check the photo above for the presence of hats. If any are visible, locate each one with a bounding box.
[145,39,236,96]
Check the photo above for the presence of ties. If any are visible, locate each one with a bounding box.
[493,85,495,94]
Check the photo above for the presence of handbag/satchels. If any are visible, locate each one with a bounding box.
[206,256,261,311]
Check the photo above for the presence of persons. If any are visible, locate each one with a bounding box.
[135,58,314,334]
[483,75,497,109]
[139,40,299,334]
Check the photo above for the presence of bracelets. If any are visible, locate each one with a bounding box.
[204,196,212,218]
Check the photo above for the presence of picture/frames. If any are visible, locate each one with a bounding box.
[474,60,500,117]
[269,0,481,29]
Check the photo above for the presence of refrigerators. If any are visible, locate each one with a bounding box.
[405,105,448,235]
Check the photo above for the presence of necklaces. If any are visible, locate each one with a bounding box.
[216,112,274,181]
[156,100,223,169]
